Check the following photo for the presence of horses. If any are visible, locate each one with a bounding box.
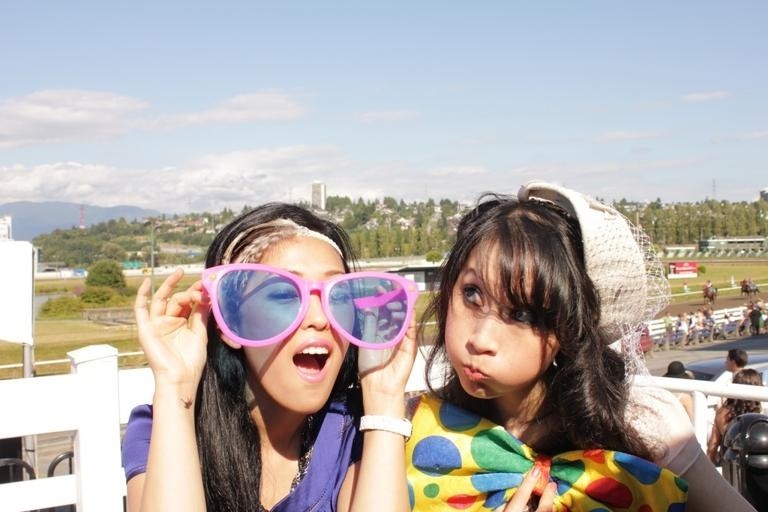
[739,282,761,298]
[702,285,718,305]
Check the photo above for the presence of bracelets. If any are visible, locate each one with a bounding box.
[357,414,412,442]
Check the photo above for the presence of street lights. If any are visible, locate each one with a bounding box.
[141,218,163,296]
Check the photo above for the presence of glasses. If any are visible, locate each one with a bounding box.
[201,262,419,350]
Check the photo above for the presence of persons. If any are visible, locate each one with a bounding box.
[120,201,418,512]
[658,276,768,489]
[404,179,757,511]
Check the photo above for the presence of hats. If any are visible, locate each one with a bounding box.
[519,181,649,345]
